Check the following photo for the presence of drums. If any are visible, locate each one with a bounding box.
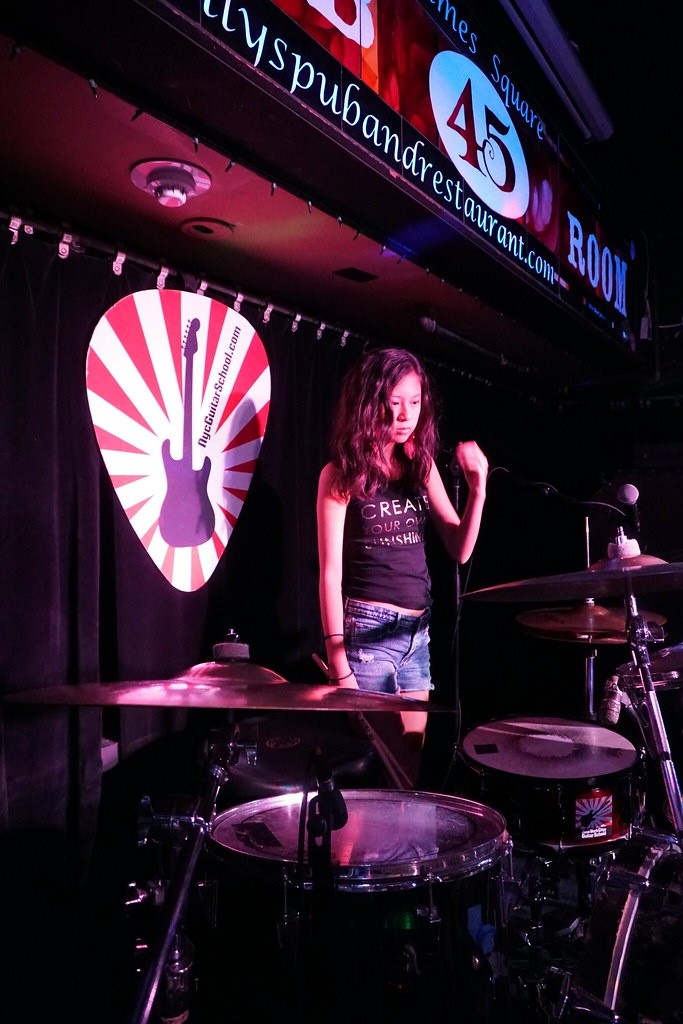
[453,715,647,853]
[506,826,683,1024]
[199,784,516,1023]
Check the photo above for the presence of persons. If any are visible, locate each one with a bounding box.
[317,347,488,789]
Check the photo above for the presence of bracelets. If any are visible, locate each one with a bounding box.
[323,634,345,640]
[329,670,354,682]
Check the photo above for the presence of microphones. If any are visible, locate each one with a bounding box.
[599,675,623,725]
[309,746,347,830]
[615,483,642,546]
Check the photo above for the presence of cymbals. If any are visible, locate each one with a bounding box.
[460,549,682,606]
[518,603,670,649]
[49,656,439,713]
